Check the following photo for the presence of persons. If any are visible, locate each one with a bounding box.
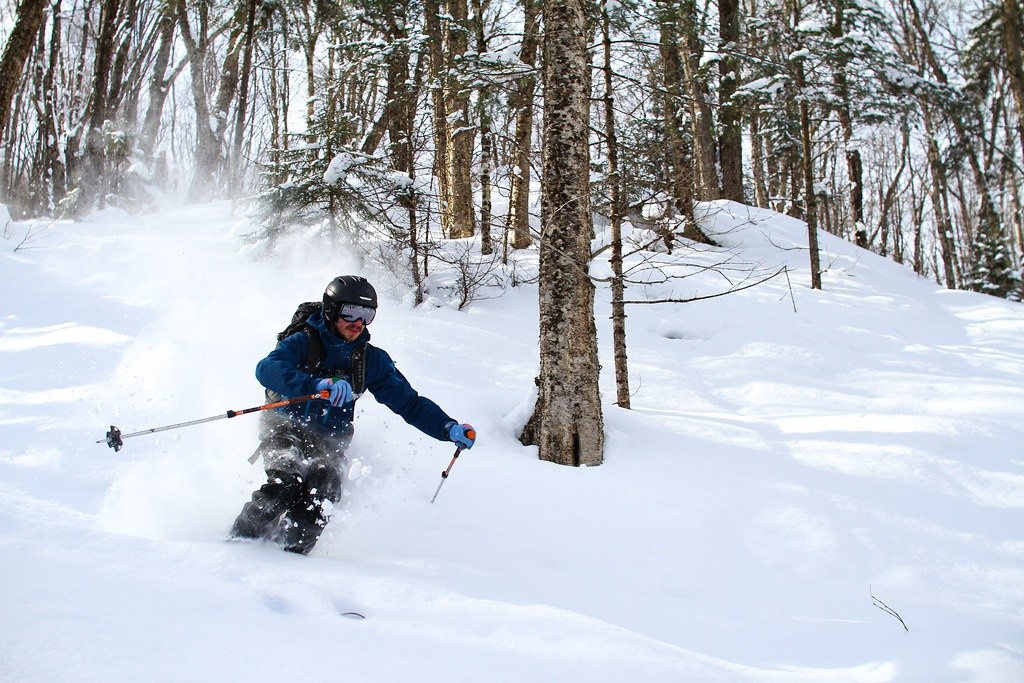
[229,275,476,556]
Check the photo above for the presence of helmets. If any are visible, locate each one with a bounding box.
[322,276,377,316]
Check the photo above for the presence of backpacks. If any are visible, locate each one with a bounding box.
[274,303,365,396]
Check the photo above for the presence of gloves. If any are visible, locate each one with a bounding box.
[316,378,354,407]
[449,424,476,450]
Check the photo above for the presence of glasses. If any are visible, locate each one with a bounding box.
[338,304,377,325]
